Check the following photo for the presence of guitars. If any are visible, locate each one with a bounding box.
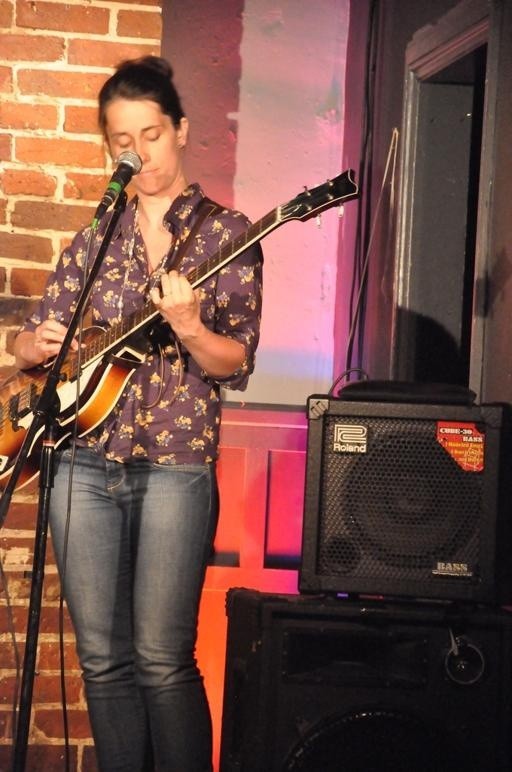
[0,168,358,496]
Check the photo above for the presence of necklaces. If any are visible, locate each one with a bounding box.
[117,199,173,323]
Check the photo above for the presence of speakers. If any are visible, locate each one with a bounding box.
[218,586,511,771]
[297,393,510,606]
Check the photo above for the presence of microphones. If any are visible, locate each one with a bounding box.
[90,151,142,229]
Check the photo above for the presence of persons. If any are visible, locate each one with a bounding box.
[14,57,265,772]
